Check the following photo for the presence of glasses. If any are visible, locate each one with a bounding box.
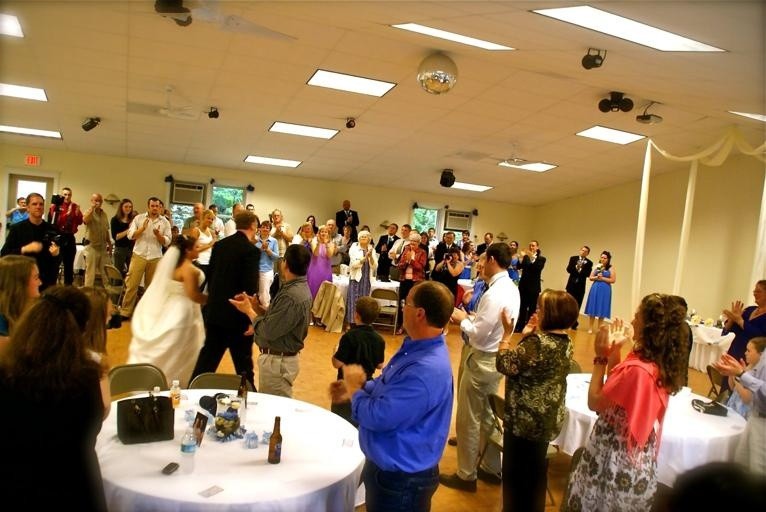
[400,300,419,310]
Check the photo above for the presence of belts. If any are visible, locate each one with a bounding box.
[258,347,296,357]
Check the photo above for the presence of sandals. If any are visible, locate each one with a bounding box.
[395,327,404,336]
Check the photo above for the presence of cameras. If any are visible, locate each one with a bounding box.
[50,194,65,204]
[96,202,100,206]
[446,252,453,260]
[40,230,61,249]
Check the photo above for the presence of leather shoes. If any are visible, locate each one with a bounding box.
[309,320,322,326]
[107,313,128,322]
[475,467,501,486]
[447,435,456,444]
[440,471,479,492]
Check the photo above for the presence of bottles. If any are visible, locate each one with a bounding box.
[153,386,161,396]
[268,416,282,463]
[237,372,248,409]
[170,380,181,409]
[180,427,198,475]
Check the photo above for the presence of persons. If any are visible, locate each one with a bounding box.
[559,291,694,510]
[709,352,766,477]
[327,280,456,511]
[437,241,523,493]
[374,223,546,335]
[0,283,108,510]
[654,458,765,511]
[564,246,593,330]
[726,337,766,419]
[584,251,616,333]
[82,193,178,322]
[721,278,766,395]
[494,287,581,511]
[129,231,210,391]
[188,213,263,393]
[294,200,379,332]
[1,186,85,336]
[182,204,293,308]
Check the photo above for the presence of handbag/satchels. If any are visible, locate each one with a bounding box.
[117,396,175,444]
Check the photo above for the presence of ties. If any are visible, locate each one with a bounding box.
[389,237,392,245]
[446,244,450,250]
[346,209,349,217]
[395,241,407,264]
[465,283,487,344]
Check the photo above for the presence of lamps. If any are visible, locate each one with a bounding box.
[580,46,609,71]
[79,116,101,132]
[440,168,456,188]
[104,194,119,206]
[344,117,356,128]
[202,106,220,119]
[596,91,634,114]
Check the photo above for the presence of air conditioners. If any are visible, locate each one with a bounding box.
[446,211,470,229]
[172,184,204,205]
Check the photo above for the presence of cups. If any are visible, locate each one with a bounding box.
[261,430,272,443]
[244,430,259,448]
[214,393,240,438]
[184,409,194,423]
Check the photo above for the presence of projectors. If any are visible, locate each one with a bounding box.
[636,114,663,126]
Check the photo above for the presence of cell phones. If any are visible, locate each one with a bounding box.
[163,463,179,473]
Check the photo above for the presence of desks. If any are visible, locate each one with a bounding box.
[331,273,401,306]
[94,388,367,511]
[685,319,737,374]
[548,372,748,489]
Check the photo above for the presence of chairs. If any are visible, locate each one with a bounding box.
[477,391,557,507]
[369,288,399,336]
[104,263,125,310]
[109,361,169,396]
[186,371,255,393]
[312,281,345,326]
[705,363,723,398]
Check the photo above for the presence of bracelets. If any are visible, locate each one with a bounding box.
[737,369,747,378]
[499,340,511,346]
[593,355,609,369]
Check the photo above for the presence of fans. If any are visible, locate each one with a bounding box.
[125,85,200,121]
[489,142,560,166]
